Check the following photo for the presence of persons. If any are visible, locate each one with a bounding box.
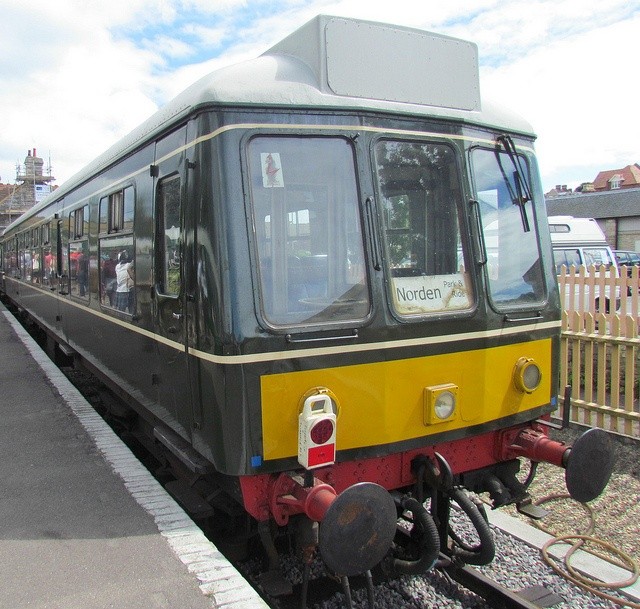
[113,250,135,313]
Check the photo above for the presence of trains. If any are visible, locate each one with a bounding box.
[0,15,615,609]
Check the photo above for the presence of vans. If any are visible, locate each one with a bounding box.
[547,215,621,313]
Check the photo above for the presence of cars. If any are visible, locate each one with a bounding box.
[615,249,640,274]
